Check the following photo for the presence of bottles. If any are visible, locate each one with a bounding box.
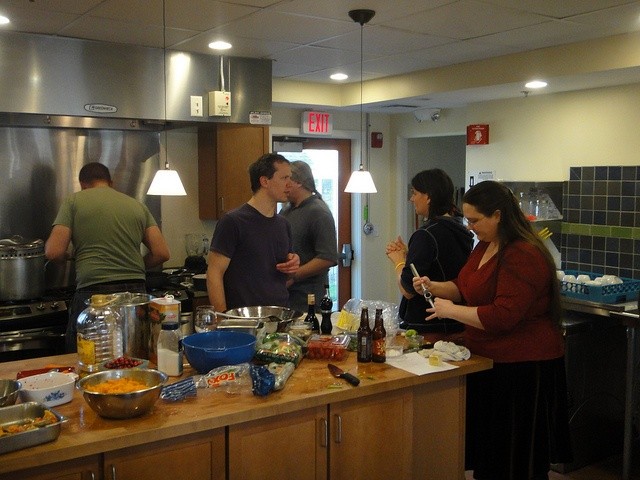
[157,321,184,376]
[320,284,333,334]
[372,308,387,363]
[357,307,372,363]
[304,293,320,334]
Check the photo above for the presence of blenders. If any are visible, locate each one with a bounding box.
[183,230,210,276]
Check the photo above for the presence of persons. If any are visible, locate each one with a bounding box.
[278,160,339,316]
[412,181,565,479]
[385,168,474,332]
[206,153,300,316]
[46,162,169,353]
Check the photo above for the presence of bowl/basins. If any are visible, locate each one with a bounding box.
[181,332,257,377]
[226,306,295,333]
[77,369,166,420]
[96,358,149,367]
[2,374,20,403]
[556,271,623,293]
[0,401,70,451]
[21,369,79,410]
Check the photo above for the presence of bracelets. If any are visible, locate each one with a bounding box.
[395,261,406,270]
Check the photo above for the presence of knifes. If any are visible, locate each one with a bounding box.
[327,361,359,386]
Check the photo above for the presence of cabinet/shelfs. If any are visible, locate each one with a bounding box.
[228,388,414,479]
[412,378,466,479]
[559,297,639,478]
[198,123,270,221]
[1,426,227,480]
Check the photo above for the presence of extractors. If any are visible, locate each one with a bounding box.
[1,27,275,132]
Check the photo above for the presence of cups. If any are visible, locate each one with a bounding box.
[517,192,549,218]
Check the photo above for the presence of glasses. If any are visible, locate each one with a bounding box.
[463,217,489,226]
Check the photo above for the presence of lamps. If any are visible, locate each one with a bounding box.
[146,0,188,197]
[340,9,379,194]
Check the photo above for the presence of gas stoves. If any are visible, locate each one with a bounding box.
[0,298,69,321]
[152,286,203,303]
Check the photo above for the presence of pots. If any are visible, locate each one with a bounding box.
[85,291,159,360]
[1,236,49,302]
